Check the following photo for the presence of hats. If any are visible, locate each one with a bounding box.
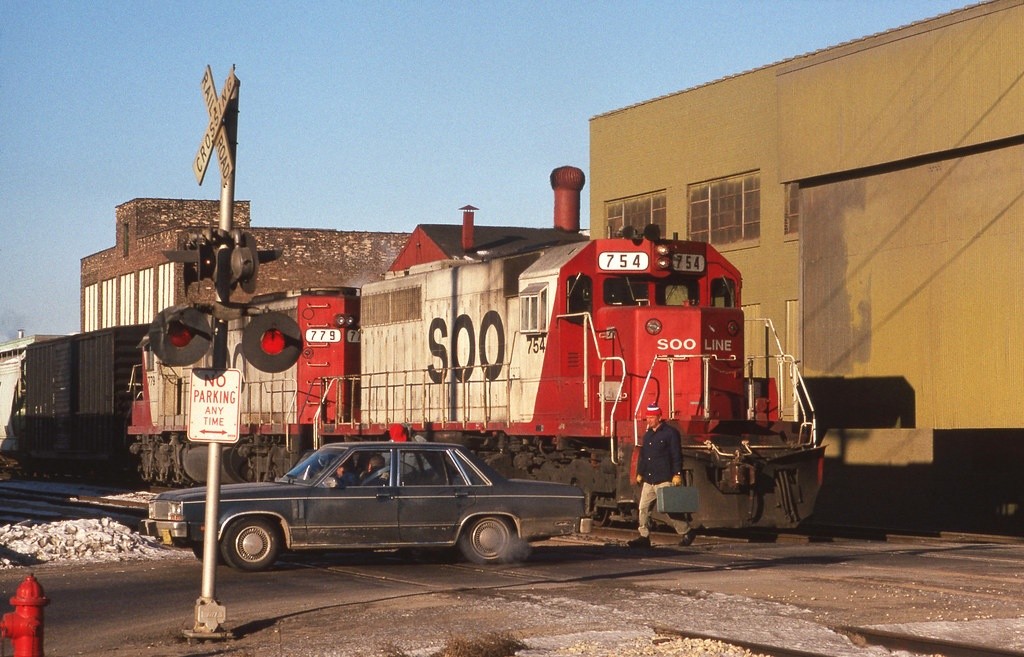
[646,405,662,416]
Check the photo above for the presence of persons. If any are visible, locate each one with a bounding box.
[627,402,698,549]
[328,453,389,489]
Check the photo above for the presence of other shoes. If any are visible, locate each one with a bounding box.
[678,529,696,546]
[627,536,651,548]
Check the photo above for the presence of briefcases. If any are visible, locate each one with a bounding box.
[656,483,697,513]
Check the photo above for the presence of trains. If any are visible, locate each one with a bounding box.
[0,165,831,541]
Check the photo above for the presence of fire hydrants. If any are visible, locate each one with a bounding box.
[1,572,51,657]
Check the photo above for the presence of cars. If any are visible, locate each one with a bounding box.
[144,440,588,574]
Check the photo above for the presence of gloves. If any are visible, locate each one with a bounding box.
[671,475,682,486]
